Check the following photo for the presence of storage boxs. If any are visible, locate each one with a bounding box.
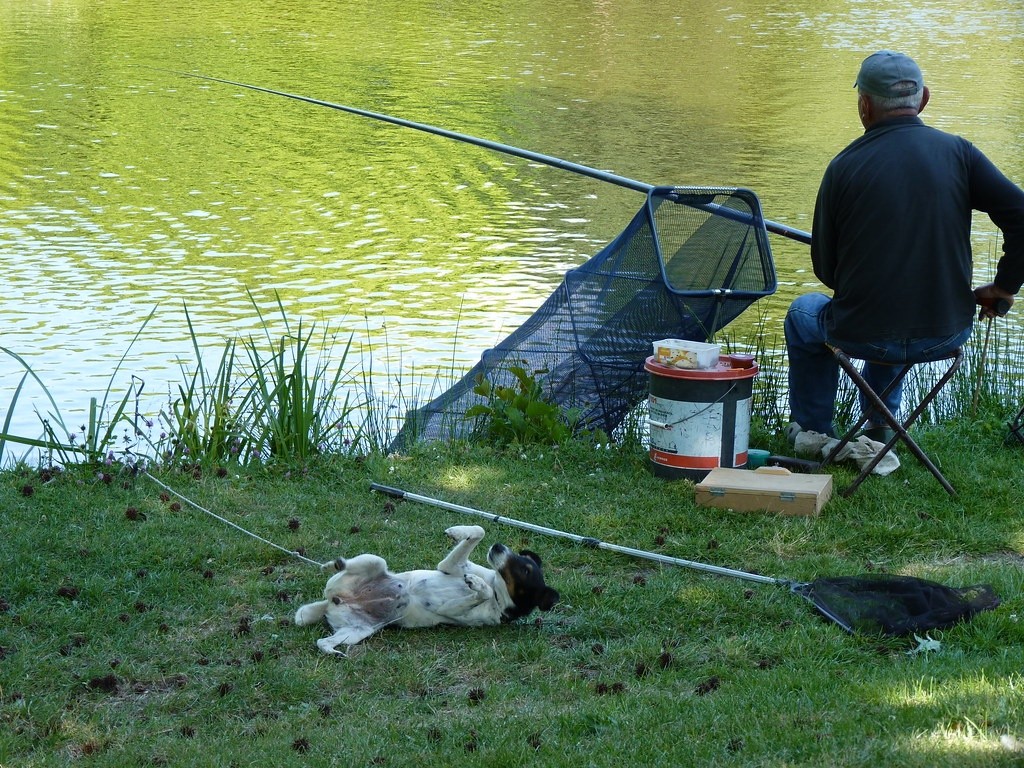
[696,467,832,517]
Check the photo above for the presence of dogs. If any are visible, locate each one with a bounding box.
[295,525,560,658]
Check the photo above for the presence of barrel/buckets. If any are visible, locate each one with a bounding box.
[644,354,760,480]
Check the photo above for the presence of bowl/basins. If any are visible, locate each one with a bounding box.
[652,338,721,369]
[729,354,755,369]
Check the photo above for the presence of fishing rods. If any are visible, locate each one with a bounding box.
[145,63,1010,315]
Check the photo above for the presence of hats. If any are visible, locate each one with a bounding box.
[853,50,924,97]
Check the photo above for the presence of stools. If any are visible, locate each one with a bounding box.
[824,343,965,504]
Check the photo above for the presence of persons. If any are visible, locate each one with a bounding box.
[778,50,1024,442]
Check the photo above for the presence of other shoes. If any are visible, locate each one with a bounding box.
[853,427,897,452]
[788,427,838,445]
